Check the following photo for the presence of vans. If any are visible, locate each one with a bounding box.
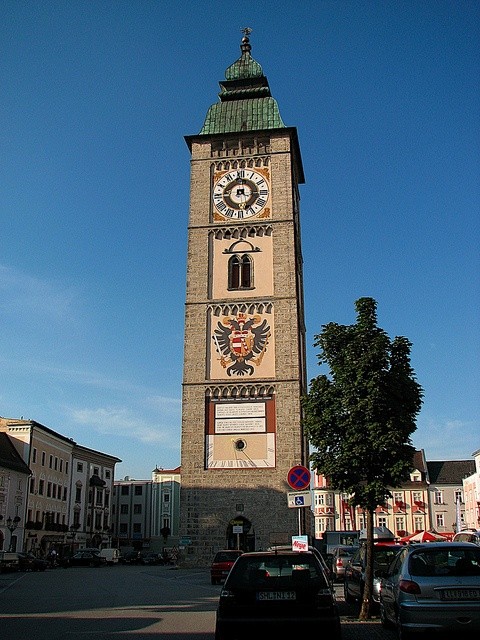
[77,548,101,559]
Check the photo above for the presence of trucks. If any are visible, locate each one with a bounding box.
[98,548,120,564]
[163,545,176,559]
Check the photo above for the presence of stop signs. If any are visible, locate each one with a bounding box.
[286,465,311,491]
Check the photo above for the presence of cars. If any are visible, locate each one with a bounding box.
[332,545,361,582]
[122,551,143,565]
[215,541,342,640]
[20,557,44,572]
[211,549,244,586]
[17,551,37,559]
[344,544,405,604]
[143,553,165,566]
[268,546,331,581]
[63,551,98,568]
[380,542,480,639]
[0,552,27,572]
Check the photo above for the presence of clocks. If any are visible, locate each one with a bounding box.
[213,169,268,222]
